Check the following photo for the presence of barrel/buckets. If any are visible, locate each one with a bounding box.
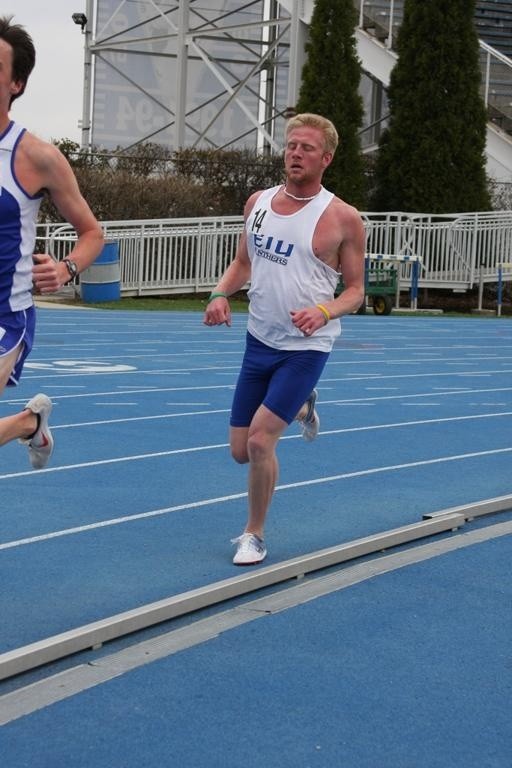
[80,242,121,304]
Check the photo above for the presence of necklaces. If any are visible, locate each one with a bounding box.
[284,187,316,200]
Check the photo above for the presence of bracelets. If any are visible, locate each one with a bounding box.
[316,304,330,325]
[208,293,225,303]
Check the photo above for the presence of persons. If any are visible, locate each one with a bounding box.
[0,16,104,471]
[203,112,365,566]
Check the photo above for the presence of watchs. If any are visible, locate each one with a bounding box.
[62,258,77,281]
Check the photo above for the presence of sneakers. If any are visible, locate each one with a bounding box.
[299,391,319,441]
[230,533,267,563]
[18,393,53,466]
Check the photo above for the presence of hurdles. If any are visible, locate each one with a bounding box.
[494,263,512,318]
[364,252,445,314]
